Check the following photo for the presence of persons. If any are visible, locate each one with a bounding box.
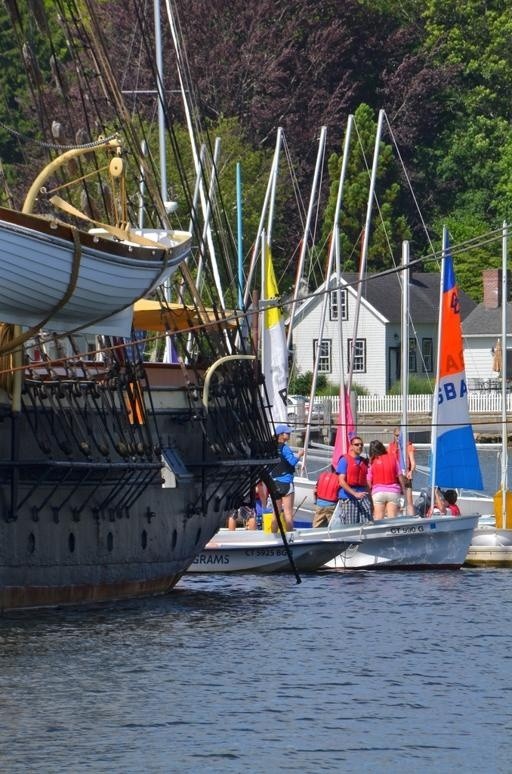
[227,423,463,531]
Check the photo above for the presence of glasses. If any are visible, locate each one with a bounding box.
[352,443,364,447]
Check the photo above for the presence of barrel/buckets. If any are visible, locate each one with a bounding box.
[261,508,287,535]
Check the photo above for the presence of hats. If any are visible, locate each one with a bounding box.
[438,488,458,504]
[275,425,292,437]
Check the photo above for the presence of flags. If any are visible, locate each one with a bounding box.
[329,386,354,469]
